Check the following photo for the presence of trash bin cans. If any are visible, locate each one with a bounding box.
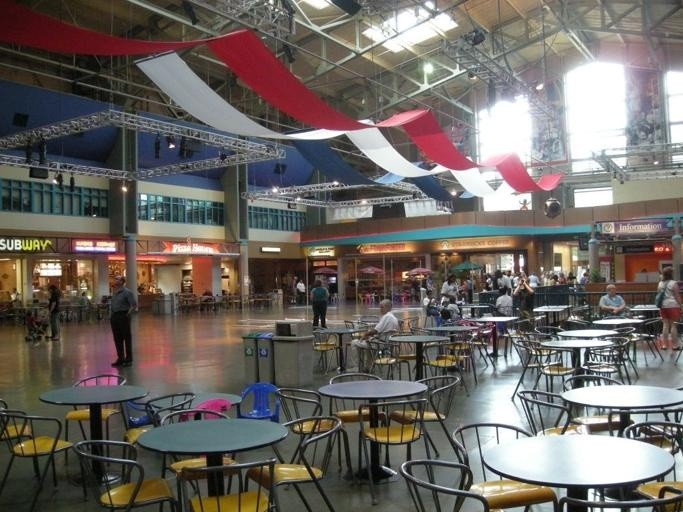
[242,332,275,385]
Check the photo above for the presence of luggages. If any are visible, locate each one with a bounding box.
[441,305,451,321]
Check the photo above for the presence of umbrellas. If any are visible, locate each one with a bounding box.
[312,267,337,277]
[356,265,383,276]
[449,261,481,278]
[405,267,435,287]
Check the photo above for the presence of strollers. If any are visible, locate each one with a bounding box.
[23,305,56,343]
[87,300,101,318]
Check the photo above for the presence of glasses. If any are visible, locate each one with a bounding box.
[430,293,432,295]
[114,277,121,281]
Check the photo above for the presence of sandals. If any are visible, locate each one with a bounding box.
[661,345,666,349]
[673,345,681,349]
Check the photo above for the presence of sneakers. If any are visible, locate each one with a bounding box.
[50,335,55,338]
[53,336,59,340]
[360,366,375,375]
[347,367,359,372]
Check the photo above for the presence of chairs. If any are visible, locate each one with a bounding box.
[365,339,401,382]
[580,343,626,388]
[0,410,88,512]
[399,316,419,332]
[529,347,579,411]
[124,401,161,432]
[386,333,416,381]
[511,340,562,400]
[344,325,375,366]
[563,375,634,433]
[316,380,428,484]
[422,342,470,398]
[557,486,683,512]
[439,322,465,344]
[533,326,566,364]
[517,308,546,333]
[672,322,683,366]
[607,407,682,482]
[638,387,682,436]
[64,373,129,465]
[121,392,197,485]
[72,440,180,512]
[175,459,281,512]
[356,398,435,506]
[236,383,280,425]
[601,316,629,329]
[0,398,36,473]
[343,320,363,341]
[312,326,339,377]
[621,421,682,512]
[328,373,392,470]
[503,306,530,332]
[518,333,562,376]
[276,388,352,478]
[570,305,591,329]
[464,325,495,369]
[311,327,338,369]
[398,460,489,512]
[158,409,244,512]
[243,415,342,512]
[588,337,639,379]
[444,331,480,387]
[178,398,231,423]
[398,320,411,352]
[495,306,514,317]
[516,390,591,438]
[565,308,587,330]
[434,337,464,383]
[409,326,441,373]
[450,423,557,512]
[632,317,663,361]
[422,305,435,329]
[606,327,636,363]
[439,308,453,323]
[387,375,460,458]
[447,318,455,323]
[359,328,397,377]
[564,319,590,330]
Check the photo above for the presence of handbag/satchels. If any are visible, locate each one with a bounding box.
[427,297,441,317]
[58,304,65,312]
[655,279,670,308]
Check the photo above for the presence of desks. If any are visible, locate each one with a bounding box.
[539,339,614,409]
[629,305,660,340]
[458,305,489,319]
[421,326,479,372]
[559,385,682,438]
[482,434,674,512]
[531,306,571,336]
[317,328,368,377]
[150,393,242,421]
[591,319,644,362]
[39,385,150,487]
[136,418,288,498]
[465,317,520,357]
[355,322,378,327]
[555,329,619,381]
[389,336,451,384]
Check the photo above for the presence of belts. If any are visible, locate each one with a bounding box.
[114,309,129,314]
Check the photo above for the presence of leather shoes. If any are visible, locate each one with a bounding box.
[121,361,132,366]
[112,359,122,366]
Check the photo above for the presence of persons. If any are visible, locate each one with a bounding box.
[425,273,434,294]
[654,265,683,350]
[26,310,38,341]
[442,274,461,299]
[447,295,460,323]
[490,287,514,317]
[110,275,137,368]
[9,287,20,308]
[467,276,473,302]
[364,285,381,303]
[512,275,535,320]
[47,284,61,341]
[78,291,93,322]
[423,291,441,326]
[392,274,427,306]
[296,279,306,304]
[202,286,214,313]
[598,283,626,320]
[309,279,329,331]
[344,298,399,373]
[477,266,590,291]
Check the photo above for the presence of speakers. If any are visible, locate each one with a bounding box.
[28,168,49,178]
